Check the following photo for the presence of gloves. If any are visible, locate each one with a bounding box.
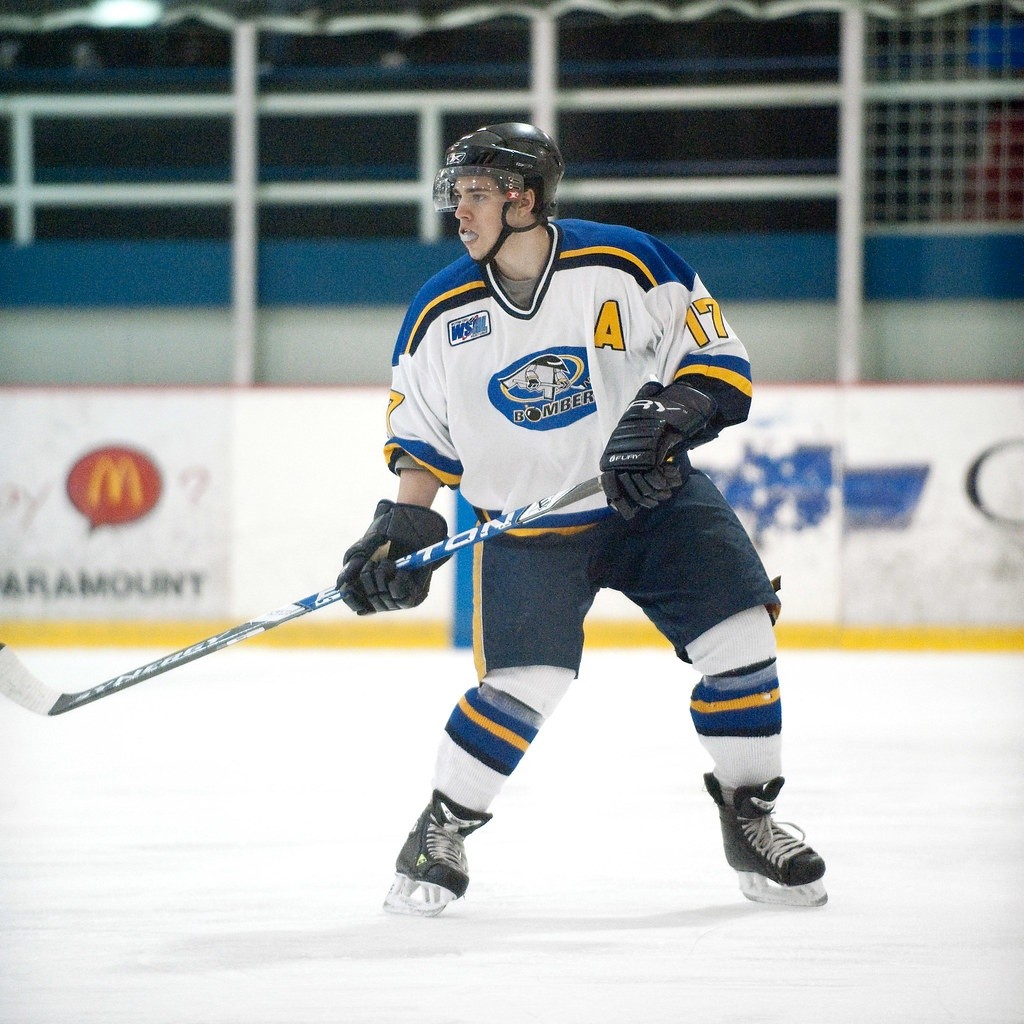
[602,383,716,522]
[337,501,447,616]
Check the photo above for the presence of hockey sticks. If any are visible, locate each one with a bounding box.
[0,467,606,717]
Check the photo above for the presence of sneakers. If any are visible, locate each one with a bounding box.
[385,791,492,917]
[702,773,829,908]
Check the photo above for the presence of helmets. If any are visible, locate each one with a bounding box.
[445,123,563,218]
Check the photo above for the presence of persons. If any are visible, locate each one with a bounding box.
[336,121,827,913]
